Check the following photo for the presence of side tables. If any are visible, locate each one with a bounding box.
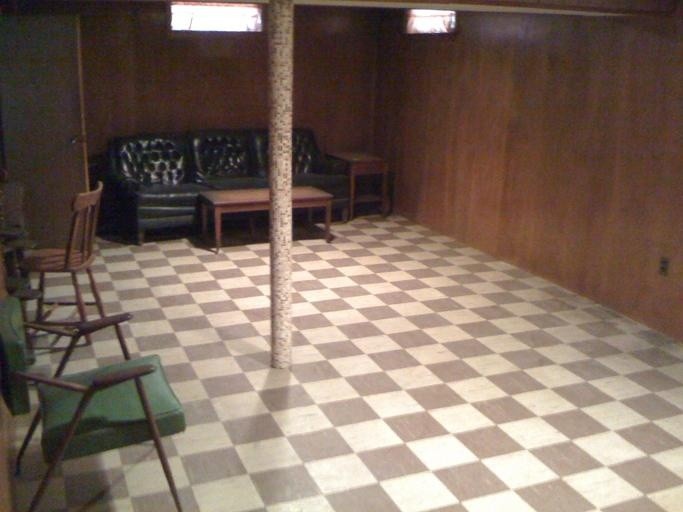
[329,151,392,219]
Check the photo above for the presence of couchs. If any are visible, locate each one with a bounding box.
[101,120,322,247]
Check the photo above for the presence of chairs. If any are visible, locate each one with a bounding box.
[2,295,192,511]
[18,186,101,317]
